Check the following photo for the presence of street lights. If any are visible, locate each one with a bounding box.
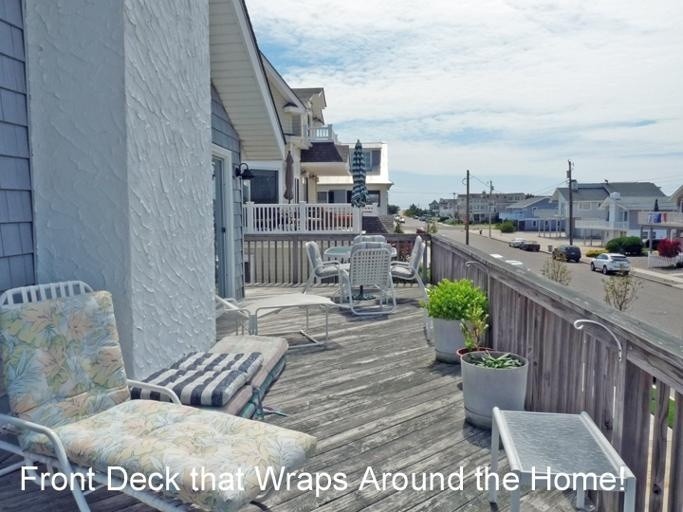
[564,158,580,247]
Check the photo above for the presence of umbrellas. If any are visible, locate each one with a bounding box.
[348,138,376,303]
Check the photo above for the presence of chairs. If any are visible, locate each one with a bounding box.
[129,333,290,422]
[302,240,349,303]
[385,235,426,305]
[214,292,336,351]
[339,242,397,316]
[0,282,318,512]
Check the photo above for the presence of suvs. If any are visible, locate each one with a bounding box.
[590,253,631,274]
[552,245,581,262]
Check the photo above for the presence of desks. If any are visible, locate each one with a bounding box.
[324,246,397,267]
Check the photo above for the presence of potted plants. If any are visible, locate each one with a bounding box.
[419,279,489,364]
[460,351,528,430]
[456,302,493,362]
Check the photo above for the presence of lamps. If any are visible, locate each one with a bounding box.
[235,162,255,180]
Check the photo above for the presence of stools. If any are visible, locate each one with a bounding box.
[488,407,636,511]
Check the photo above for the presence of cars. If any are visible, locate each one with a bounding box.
[519,240,540,252]
[509,238,526,248]
